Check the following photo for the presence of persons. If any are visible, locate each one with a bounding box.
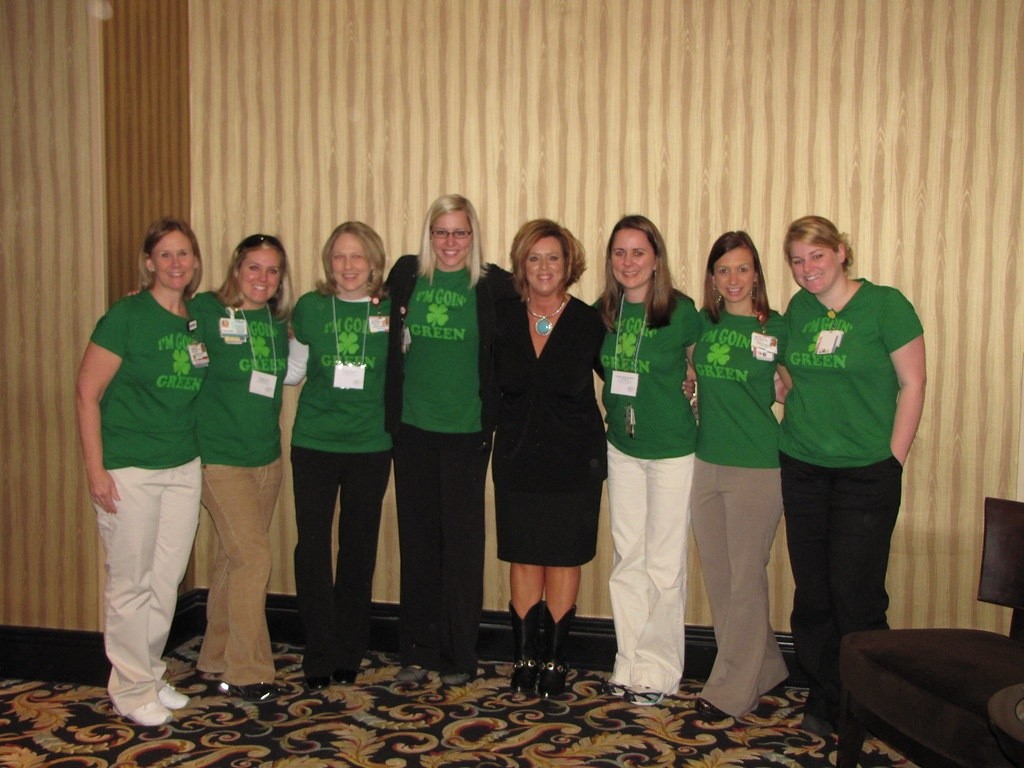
[778,215,927,738]
[285,221,404,689]
[74,214,211,726]
[485,219,695,700]
[685,230,791,722]
[126,233,289,704]
[591,215,789,706]
[383,194,499,686]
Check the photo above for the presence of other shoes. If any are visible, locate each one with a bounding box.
[802,713,833,736]
[624,685,667,706]
[695,697,728,721]
[396,665,428,682]
[439,664,471,684]
[599,682,626,699]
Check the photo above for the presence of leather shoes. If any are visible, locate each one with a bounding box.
[304,676,330,689]
[217,680,280,703]
[335,668,356,684]
[196,669,223,680]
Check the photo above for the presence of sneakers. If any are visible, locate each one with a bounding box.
[112,701,173,725]
[157,683,190,709]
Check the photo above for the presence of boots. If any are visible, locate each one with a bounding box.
[509,599,542,691]
[538,603,576,696]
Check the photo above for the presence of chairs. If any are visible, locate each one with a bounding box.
[834,499,1023,768]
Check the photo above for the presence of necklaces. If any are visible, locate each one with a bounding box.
[527,296,566,336]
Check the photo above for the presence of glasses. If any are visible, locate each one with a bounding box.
[431,230,472,239]
[238,234,278,256]
